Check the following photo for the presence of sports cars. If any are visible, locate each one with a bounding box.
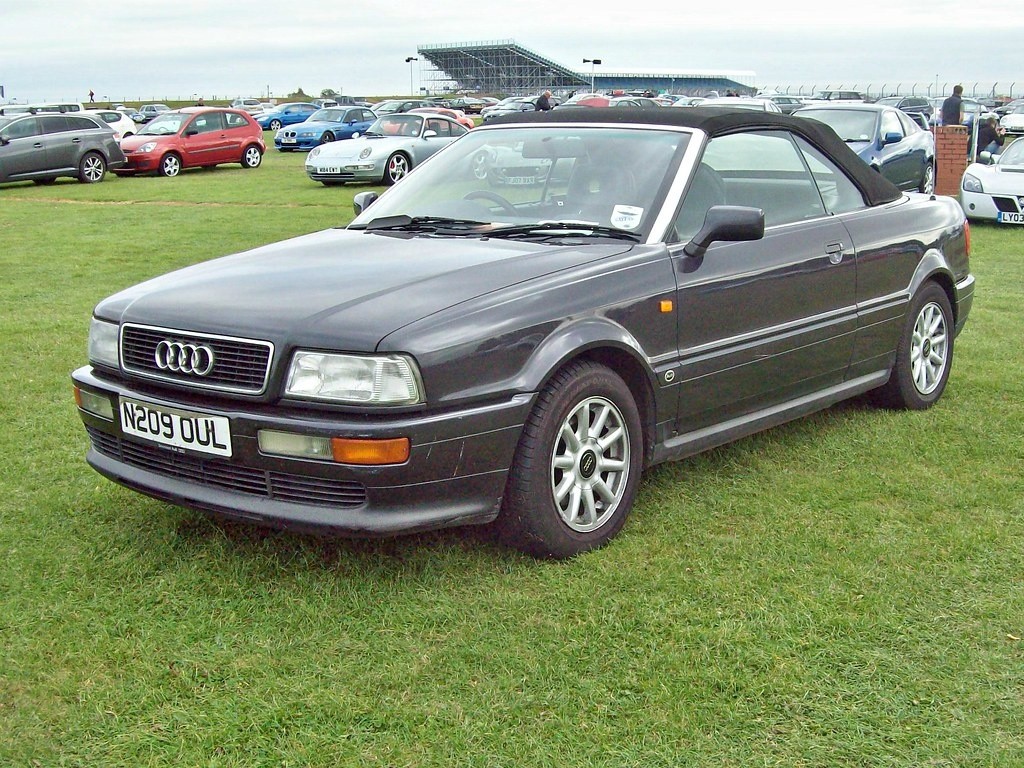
[959,136,1024,223]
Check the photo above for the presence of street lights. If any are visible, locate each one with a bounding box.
[405,56,418,95]
[583,59,602,95]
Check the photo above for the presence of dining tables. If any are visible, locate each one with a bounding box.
[330,112,341,121]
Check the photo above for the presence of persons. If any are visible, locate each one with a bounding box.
[967,116,1007,165]
[535,91,552,111]
[726,90,739,97]
[940,85,965,126]
[88,90,94,103]
[195,98,205,107]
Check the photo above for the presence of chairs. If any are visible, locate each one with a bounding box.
[564,156,637,221]
[640,161,729,242]
[402,121,442,137]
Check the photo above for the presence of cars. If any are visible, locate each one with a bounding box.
[71,107,975,561]
[226,91,1024,206]
[0,101,265,187]
